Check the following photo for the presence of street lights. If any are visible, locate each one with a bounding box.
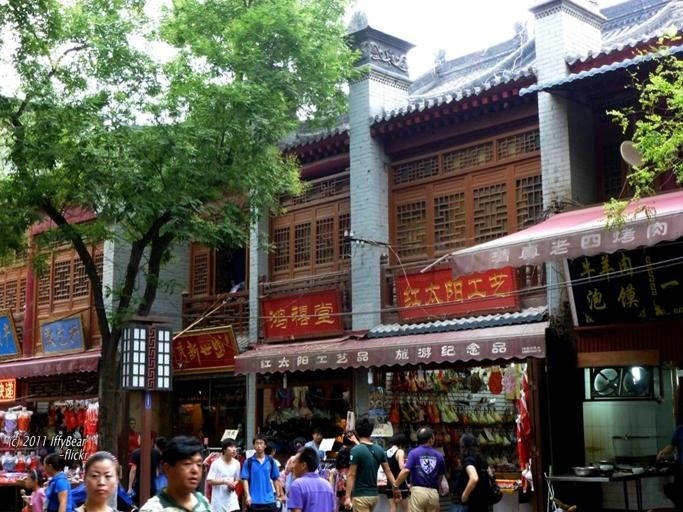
[120,314,173,505]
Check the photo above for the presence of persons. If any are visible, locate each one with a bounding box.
[0,407,501,512]
[655,424,682,510]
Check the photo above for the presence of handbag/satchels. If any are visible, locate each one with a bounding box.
[390,394,503,426]
[477,470,502,505]
[391,369,466,394]
[369,420,393,437]
[389,428,511,448]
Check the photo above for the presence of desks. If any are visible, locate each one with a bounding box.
[544,467,673,512]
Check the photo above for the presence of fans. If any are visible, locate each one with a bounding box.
[589,366,656,401]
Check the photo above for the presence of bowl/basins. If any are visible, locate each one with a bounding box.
[571,467,599,477]
